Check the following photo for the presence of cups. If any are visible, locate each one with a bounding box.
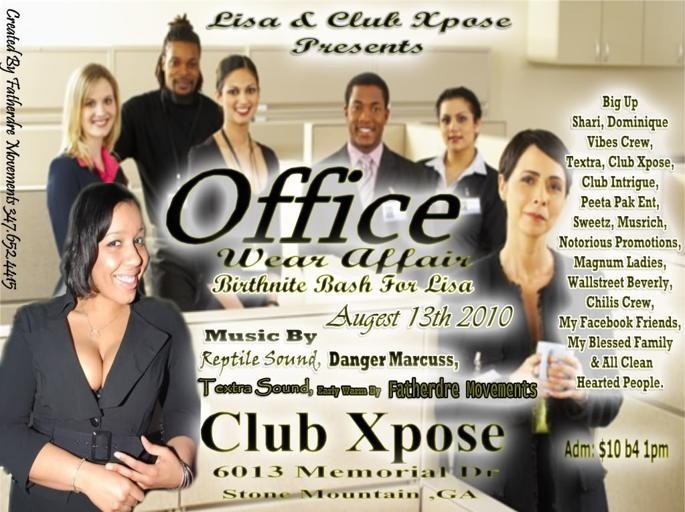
[534,341,576,395]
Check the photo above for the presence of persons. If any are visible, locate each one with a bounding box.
[1,13,624,512]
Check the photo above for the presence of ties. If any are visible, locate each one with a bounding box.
[357,156,372,206]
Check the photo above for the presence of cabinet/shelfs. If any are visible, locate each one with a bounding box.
[528,0,685,69]
